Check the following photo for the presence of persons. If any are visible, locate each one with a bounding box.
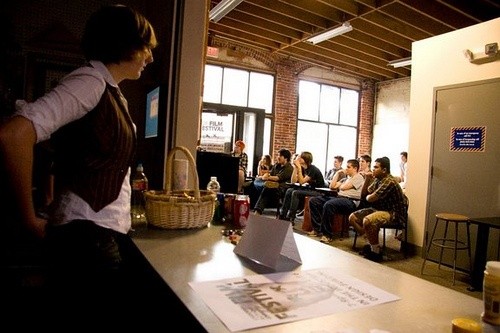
[230,141,247,193]
[0,5,158,333]
[254,149,407,258]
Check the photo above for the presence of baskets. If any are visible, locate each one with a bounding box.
[143,146,216,230]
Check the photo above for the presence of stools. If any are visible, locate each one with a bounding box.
[422,214,472,286]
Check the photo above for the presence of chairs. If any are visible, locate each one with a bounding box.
[315,174,372,237]
[276,188,284,215]
[352,194,410,251]
[285,182,311,225]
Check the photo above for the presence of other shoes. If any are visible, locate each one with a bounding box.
[359,245,383,262]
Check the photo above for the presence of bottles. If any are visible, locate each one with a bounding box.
[481,261,500,324]
[207,177,220,207]
[131,164,148,218]
[8,89,14,103]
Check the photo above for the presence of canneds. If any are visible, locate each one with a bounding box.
[224,193,236,224]
[212,192,225,225]
[233,194,250,229]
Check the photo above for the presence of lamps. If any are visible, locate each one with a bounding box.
[305,20,352,44]
[209,0,243,23]
[387,56,411,68]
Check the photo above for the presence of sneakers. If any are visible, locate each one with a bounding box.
[320,236,334,244]
[306,230,318,237]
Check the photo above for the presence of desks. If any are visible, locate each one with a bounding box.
[124,217,500,333]
[471,214,499,287]
[197,150,239,193]
